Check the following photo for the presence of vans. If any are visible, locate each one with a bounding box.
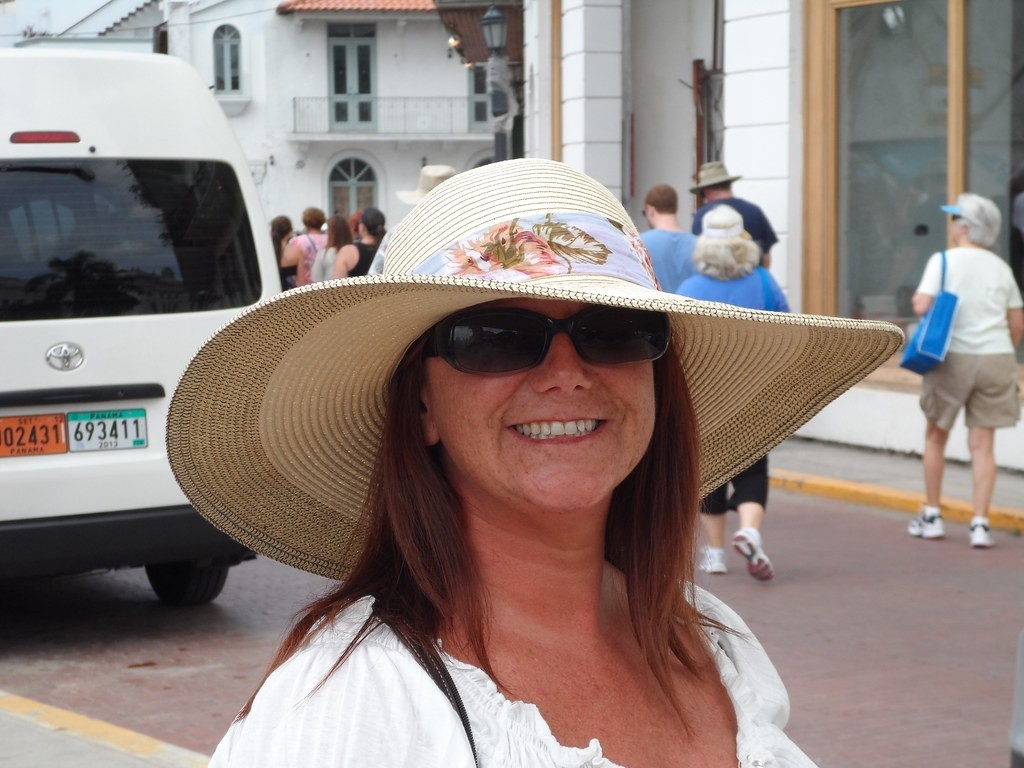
[1,51,283,607]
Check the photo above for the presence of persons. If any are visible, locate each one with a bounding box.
[167,159,906,768]
[271,207,386,292]
[909,195,1024,546]
[638,187,698,295]
[691,163,779,268]
[679,209,789,577]
[367,165,457,275]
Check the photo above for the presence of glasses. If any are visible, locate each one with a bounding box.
[425,304,672,377]
[952,214,961,222]
[642,208,649,216]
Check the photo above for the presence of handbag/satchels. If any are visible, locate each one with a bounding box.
[899,250,958,374]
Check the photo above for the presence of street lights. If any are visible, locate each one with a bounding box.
[479,4,506,163]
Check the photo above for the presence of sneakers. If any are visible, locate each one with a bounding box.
[699,550,727,573]
[969,524,994,546]
[733,531,774,581]
[908,514,946,539]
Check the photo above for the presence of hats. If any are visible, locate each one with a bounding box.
[940,205,964,218]
[165,159,906,581]
[702,224,752,240]
[397,165,458,206]
[689,162,740,196]
